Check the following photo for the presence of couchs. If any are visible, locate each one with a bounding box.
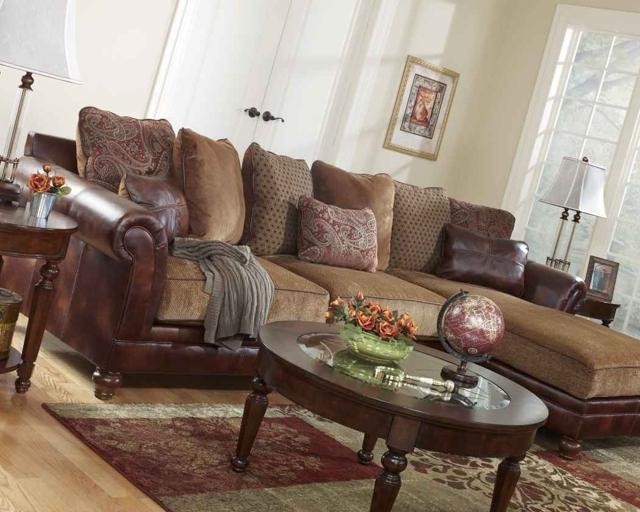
[0,128,639,462]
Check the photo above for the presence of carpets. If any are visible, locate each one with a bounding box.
[42,392,640,512]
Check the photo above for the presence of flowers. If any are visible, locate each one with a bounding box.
[28,163,72,196]
[324,291,419,340]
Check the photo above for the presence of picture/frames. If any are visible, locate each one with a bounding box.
[585,254,619,303]
[384,55,460,161]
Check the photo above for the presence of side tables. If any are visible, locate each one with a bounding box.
[576,295,621,329]
[0,193,78,392]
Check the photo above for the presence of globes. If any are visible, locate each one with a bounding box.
[434,288,504,388]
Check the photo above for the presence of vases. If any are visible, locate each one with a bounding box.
[29,192,56,219]
[343,322,417,364]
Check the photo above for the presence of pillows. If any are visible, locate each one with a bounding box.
[392,180,451,274]
[241,141,314,255]
[297,192,378,275]
[118,171,188,242]
[76,107,177,194]
[173,127,246,243]
[431,220,528,292]
[311,158,394,273]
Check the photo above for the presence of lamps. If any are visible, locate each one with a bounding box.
[0,0,85,207]
[538,155,607,272]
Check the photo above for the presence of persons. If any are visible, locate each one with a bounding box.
[596,268,611,292]
